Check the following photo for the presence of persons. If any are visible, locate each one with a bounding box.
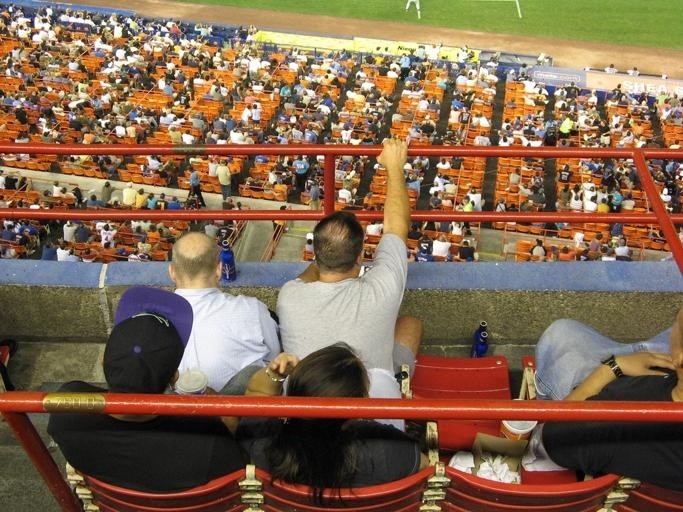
[239,340,430,510]
[273,136,424,387]
[157,231,284,394]
[0,0,683,264]
[44,284,247,510]
[530,296,683,491]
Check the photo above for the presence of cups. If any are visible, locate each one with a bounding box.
[175,368,208,395]
[499,398,538,441]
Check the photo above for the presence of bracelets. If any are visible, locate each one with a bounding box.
[599,350,624,380]
[266,365,287,382]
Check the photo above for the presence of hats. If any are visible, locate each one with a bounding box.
[103,288,193,394]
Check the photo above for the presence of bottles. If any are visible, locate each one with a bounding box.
[471,320,489,357]
[220,239,237,282]
